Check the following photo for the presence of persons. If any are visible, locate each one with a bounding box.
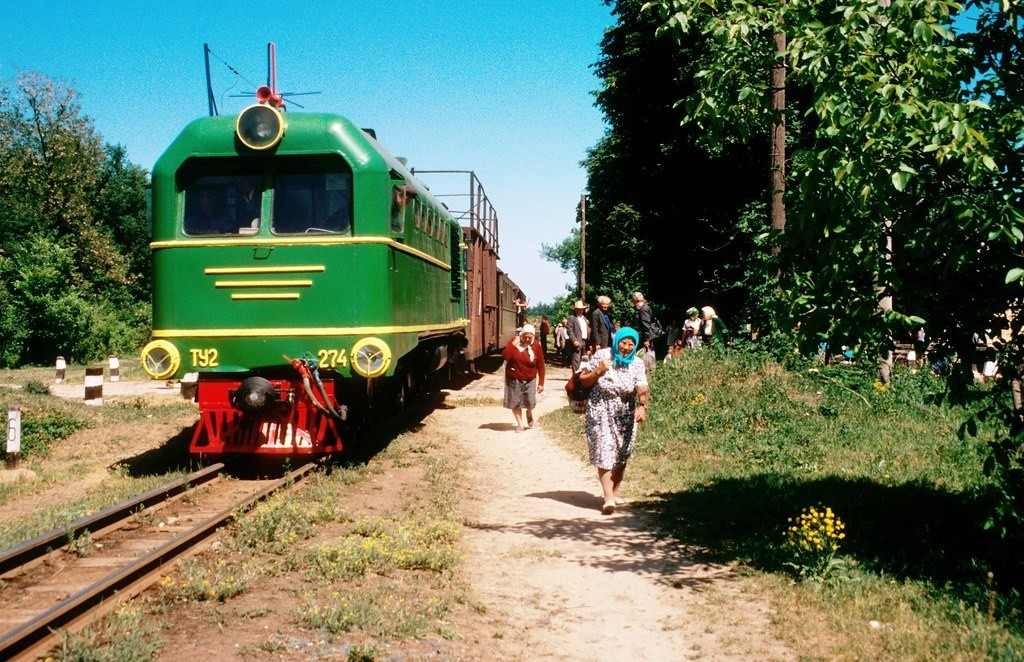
[914,325,926,369]
[701,306,731,351]
[515,297,683,365]
[682,306,701,354]
[502,324,546,433]
[579,326,649,515]
[567,300,591,374]
[811,332,894,383]
[591,296,612,351]
[631,291,653,365]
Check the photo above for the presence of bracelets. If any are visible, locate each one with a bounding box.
[639,402,647,410]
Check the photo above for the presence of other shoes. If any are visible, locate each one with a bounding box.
[516,426,524,433]
[603,501,615,513]
[526,411,534,426]
[615,498,624,504]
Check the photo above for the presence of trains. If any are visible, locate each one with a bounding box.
[143,45,528,457]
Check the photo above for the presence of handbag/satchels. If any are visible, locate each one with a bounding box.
[565,349,609,414]
[642,345,656,370]
[640,304,665,341]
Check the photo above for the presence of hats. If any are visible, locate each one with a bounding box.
[572,301,587,310]
